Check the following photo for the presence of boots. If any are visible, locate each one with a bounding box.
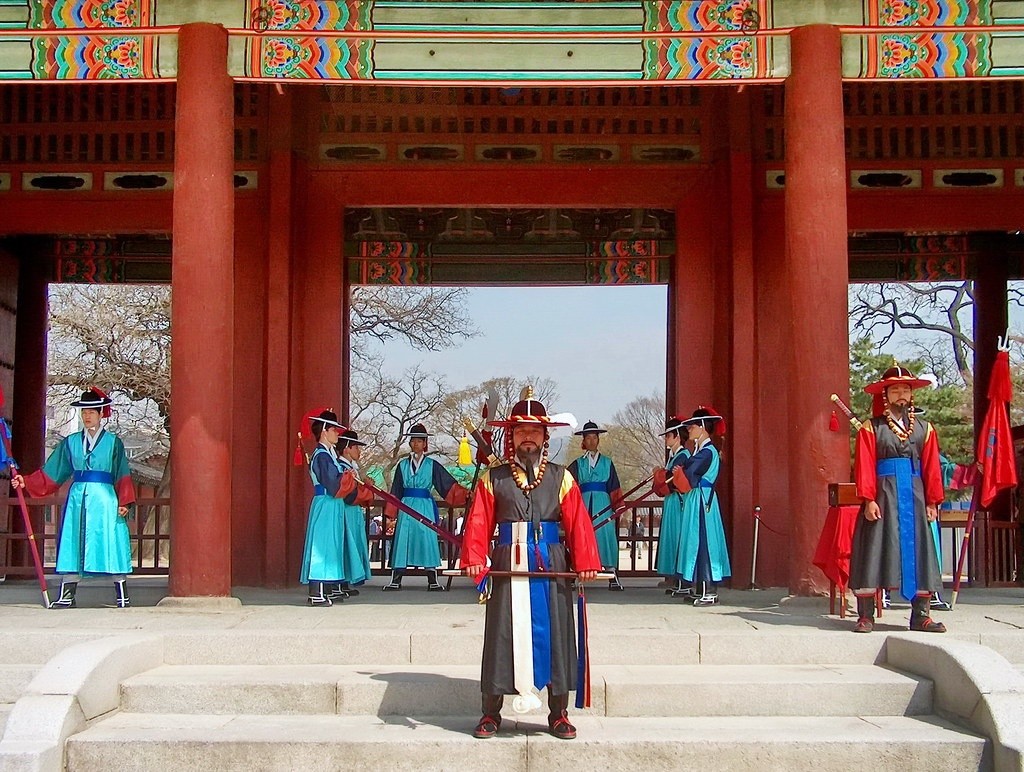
[475,693,504,737]
[569,568,576,590]
[383,570,402,590]
[114,580,130,607]
[49,582,77,607]
[548,687,576,739]
[854,595,874,632]
[665,577,719,606]
[308,581,358,606]
[426,569,445,591]
[606,568,624,591]
[910,595,946,632]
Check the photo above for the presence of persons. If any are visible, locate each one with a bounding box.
[848,357,984,633]
[652,403,731,606]
[10,386,135,608]
[459,385,601,739]
[566,420,625,592]
[299,410,473,608]
[628,516,645,559]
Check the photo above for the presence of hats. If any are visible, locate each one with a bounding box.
[575,419,607,435]
[338,428,366,445]
[658,416,687,436]
[864,358,932,395]
[402,424,434,437]
[486,386,569,426]
[681,406,722,426]
[70,387,113,408]
[907,406,926,415]
[308,408,347,429]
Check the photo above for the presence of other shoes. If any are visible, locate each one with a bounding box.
[882,590,892,609]
[930,592,950,610]
